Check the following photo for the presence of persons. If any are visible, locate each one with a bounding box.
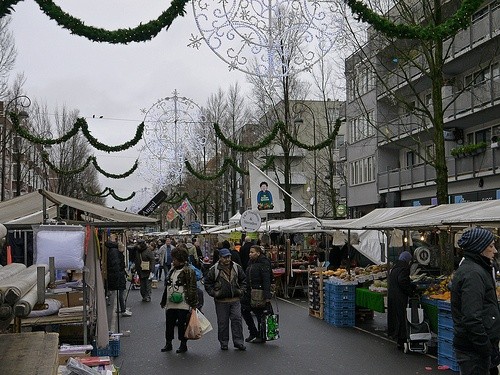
[127,233,252,289]
[449,228,500,375]
[159,247,199,354]
[203,249,248,350]
[239,244,273,344]
[104,234,135,318]
[386,250,420,351]
[134,239,156,302]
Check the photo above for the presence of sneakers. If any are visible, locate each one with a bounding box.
[251,337,266,343]
[161,344,172,352]
[176,345,187,352]
[221,343,228,351]
[245,333,258,342]
[234,343,246,350]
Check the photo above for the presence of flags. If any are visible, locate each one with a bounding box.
[165,208,179,222]
[248,163,280,215]
[176,198,193,217]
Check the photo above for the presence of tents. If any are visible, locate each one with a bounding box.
[199,199,500,269]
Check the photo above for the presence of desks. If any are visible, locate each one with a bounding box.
[9,311,90,333]
[272,267,309,298]
[0,332,60,375]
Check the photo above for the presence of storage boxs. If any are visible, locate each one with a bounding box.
[67,291,86,307]
[45,293,67,308]
[309,264,463,374]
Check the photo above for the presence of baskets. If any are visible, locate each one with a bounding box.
[93,336,119,357]
[324,280,356,327]
[437,301,461,371]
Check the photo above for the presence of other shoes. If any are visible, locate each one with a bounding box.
[142,298,145,301]
[146,296,151,302]
[125,310,132,314]
[122,311,132,317]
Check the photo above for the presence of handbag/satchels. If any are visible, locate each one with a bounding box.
[251,289,266,306]
[261,314,281,341]
[184,308,212,339]
[141,261,150,270]
[171,292,183,303]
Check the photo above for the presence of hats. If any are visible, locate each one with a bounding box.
[219,248,232,258]
[458,228,494,254]
[399,251,412,265]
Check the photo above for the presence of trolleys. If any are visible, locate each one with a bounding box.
[404,295,432,355]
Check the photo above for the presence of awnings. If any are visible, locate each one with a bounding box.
[0,188,163,235]
